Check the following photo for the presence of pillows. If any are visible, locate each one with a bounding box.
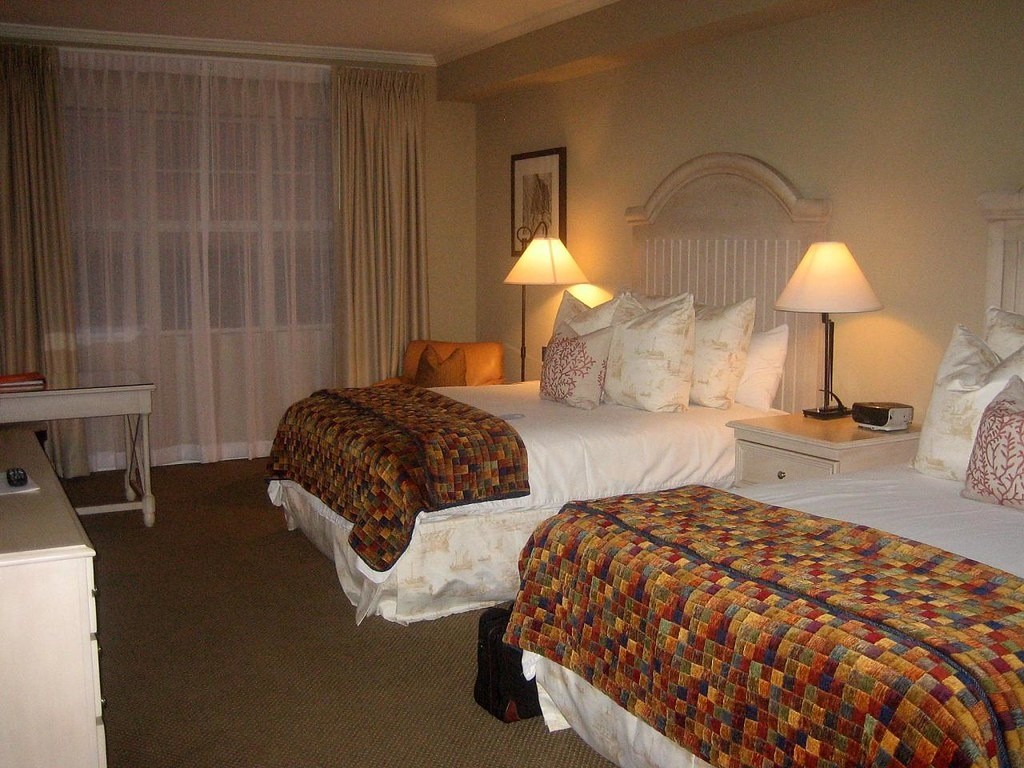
[984,306,1024,360]
[538,321,614,409]
[908,324,1024,484]
[735,323,789,413]
[554,290,620,336]
[601,290,695,413]
[415,345,467,386]
[648,296,756,410]
[959,374,1024,514]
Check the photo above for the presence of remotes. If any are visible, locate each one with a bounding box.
[7,468,28,486]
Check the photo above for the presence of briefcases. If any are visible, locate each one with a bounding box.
[473,602,543,723]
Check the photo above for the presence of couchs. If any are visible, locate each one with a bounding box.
[372,341,505,388]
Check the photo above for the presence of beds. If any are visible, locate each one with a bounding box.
[263,153,835,627]
[473,188,1024,768]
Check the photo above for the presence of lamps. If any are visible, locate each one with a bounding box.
[501,222,589,382]
[771,242,884,420]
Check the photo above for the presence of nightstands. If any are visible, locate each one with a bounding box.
[725,410,920,487]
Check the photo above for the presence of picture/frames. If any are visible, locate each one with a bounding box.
[511,147,566,257]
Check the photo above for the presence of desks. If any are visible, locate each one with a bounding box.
[0,370,156,528]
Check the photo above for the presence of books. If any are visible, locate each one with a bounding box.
[0,371,47,392]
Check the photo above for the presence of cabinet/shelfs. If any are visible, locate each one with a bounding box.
[0,429,107,768]
